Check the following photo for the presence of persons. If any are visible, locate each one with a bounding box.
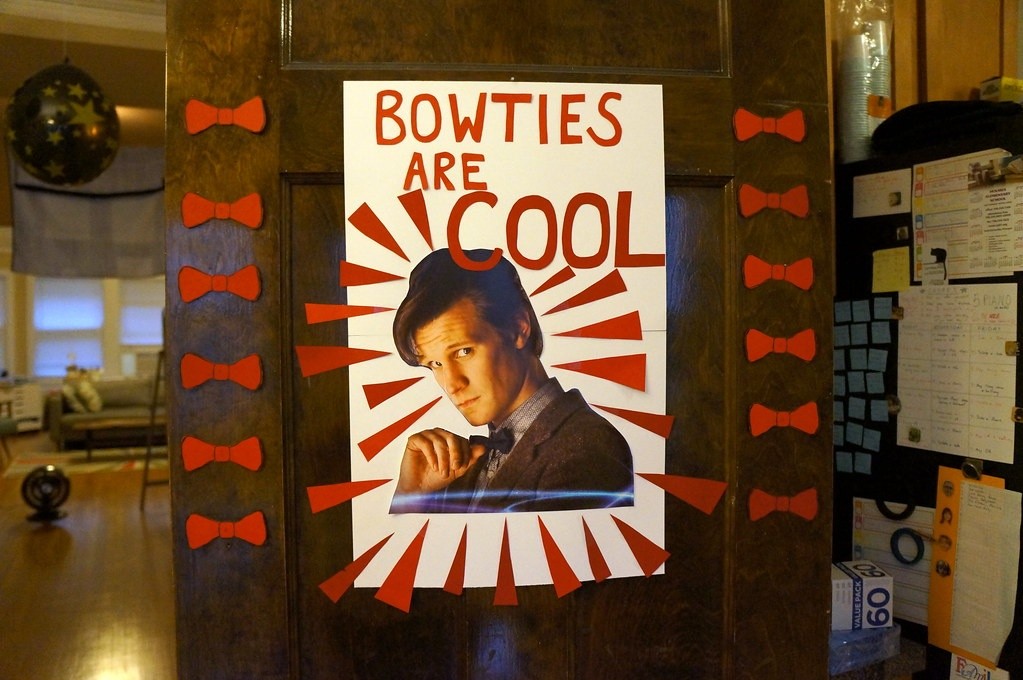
[387,248,636,513]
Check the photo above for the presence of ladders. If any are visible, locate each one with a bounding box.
[139,347,170,513]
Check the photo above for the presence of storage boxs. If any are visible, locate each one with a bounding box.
[831,560,894,629]
[981,75,1023,105]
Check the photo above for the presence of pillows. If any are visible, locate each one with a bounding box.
[78,380,102,412]
[62,384,84,412]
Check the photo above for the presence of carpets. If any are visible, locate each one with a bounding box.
[4,450,170,472]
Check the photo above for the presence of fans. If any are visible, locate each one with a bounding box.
[20,464,72,522]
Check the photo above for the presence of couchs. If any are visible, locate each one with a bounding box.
[48,377,169,449]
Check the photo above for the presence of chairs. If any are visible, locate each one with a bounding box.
[1,399,19,472]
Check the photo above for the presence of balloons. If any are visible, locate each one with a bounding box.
[0,51,121,188]
